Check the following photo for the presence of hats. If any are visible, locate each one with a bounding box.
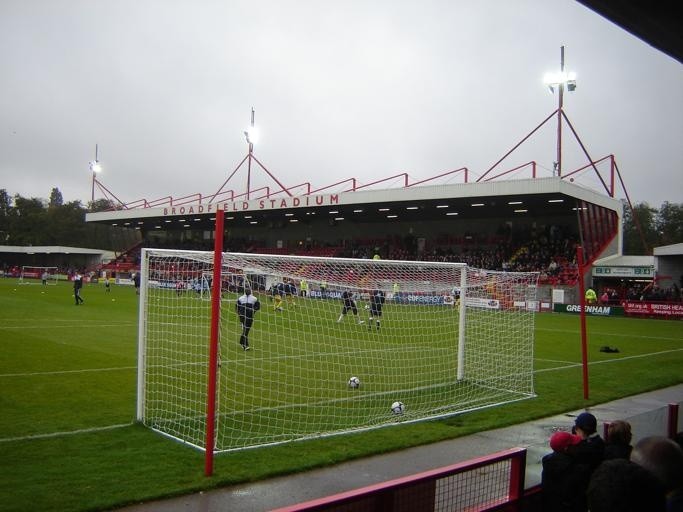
[576,412,598,435]
[551,431,580,450]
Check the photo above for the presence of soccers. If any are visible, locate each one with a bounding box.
[391,401,406,415]
[112,299,116,302]
[364,305,370,310]
[348,376,359,389]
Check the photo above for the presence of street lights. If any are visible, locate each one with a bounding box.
[241,106,261,201]
[85,140,104,210]
[542,44,576,176]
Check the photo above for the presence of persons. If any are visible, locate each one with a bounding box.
[540,412,683,512]
[234,288,260,351]
